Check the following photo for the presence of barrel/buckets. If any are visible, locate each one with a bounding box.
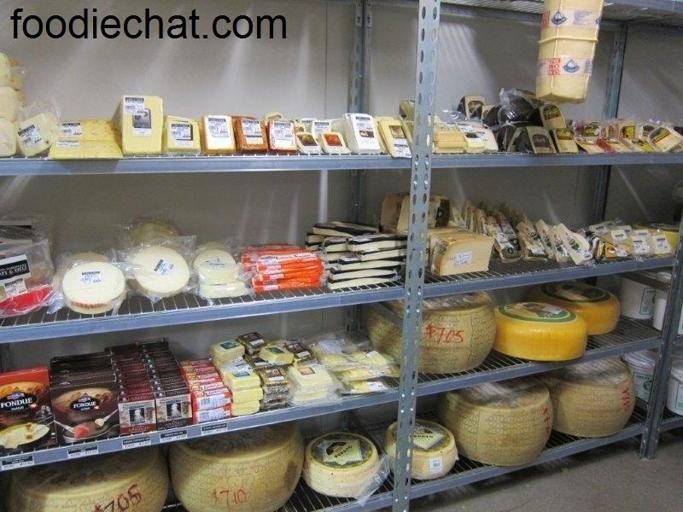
[667,338,683,416]
[624,351,657,402]
[653,271,683,335]
[619,270,659,320]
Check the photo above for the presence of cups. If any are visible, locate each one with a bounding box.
[63,422,119,444]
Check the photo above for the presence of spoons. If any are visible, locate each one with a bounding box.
[54,419,90,436]
[94,408,118,429]
